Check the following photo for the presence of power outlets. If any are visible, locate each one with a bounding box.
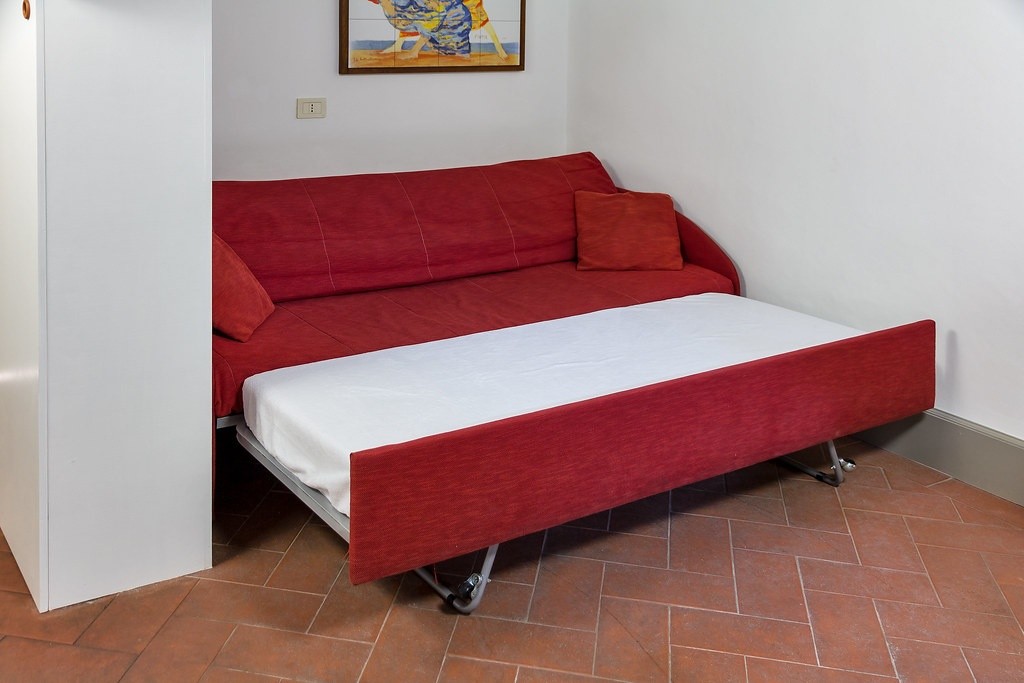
[297,98,327,119]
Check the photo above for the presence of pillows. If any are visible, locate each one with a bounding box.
[212,231,275,343]
[575,190,682,271]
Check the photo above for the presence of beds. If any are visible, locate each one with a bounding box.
[212,152,936,613]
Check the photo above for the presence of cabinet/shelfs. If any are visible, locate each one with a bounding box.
[0,0,212,613]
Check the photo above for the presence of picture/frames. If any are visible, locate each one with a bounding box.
[339,0,526,74]
